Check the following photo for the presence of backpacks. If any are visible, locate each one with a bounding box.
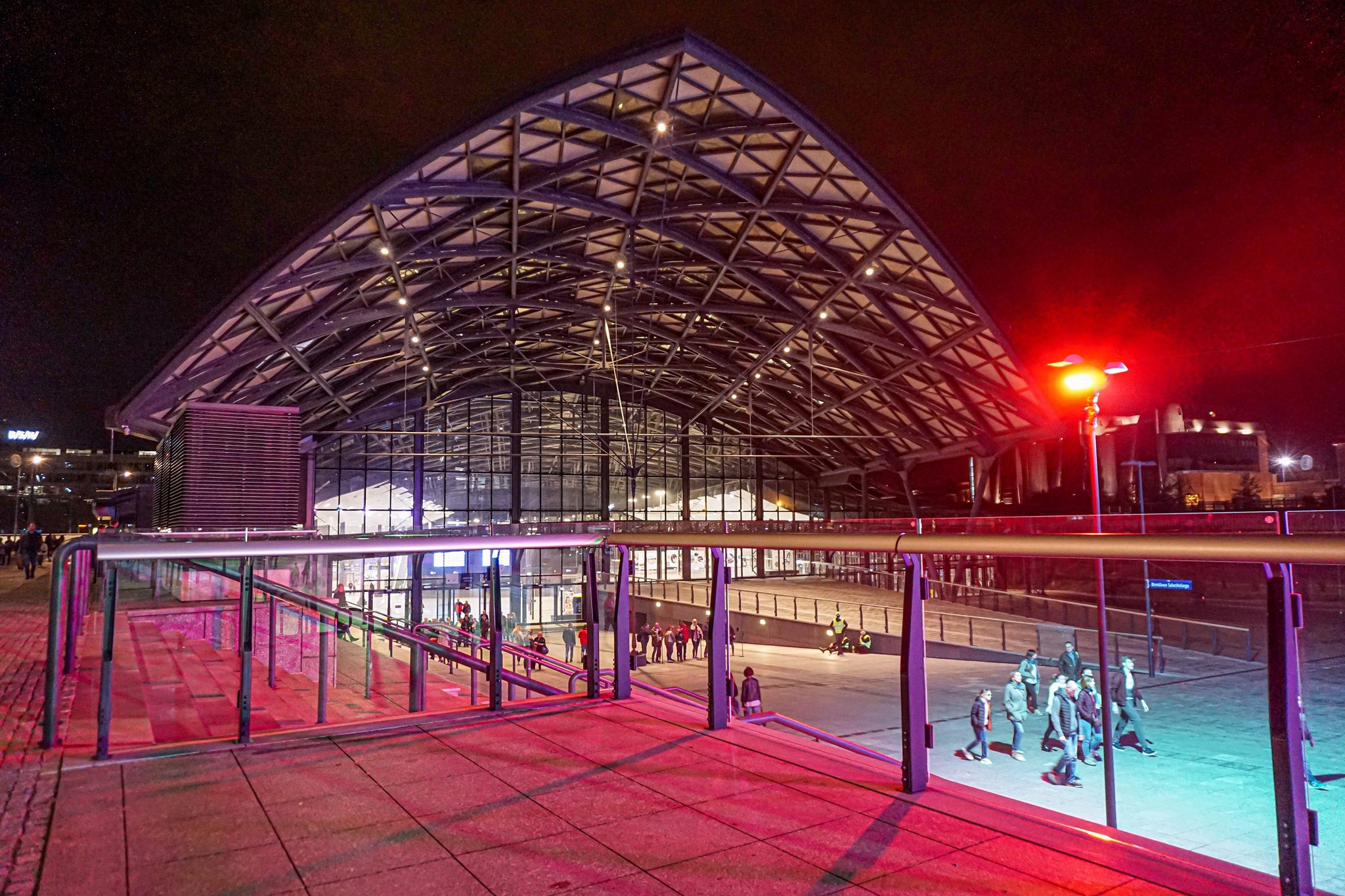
[637,634,641,642]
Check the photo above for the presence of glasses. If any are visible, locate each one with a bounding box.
[983,694,992,696]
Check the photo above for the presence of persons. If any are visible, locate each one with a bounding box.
[422,598,517,666]
[1298,694,1331,791]
[725,620,736,656]
[370,582,374,594]
[0,520,65,580]
[291,560,311,588]
[91,520,133,578]
[348,581,356,594]
[333,584,359,642]
[725,666,763,726]
[562,622,588,663]
[513,626,545,672]
[604,592,616,631]
[819,612,871,656]
[960,641,1157,788]
[639,617,710,664]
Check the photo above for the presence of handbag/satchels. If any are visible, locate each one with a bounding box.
[1034,680,1040,695]
[761,708,766,727]
[733,699,740,715]
[541,646,549,655]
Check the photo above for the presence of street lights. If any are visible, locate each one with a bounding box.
[1046,354,1127,829]
[1281,456,1291,499]
[31,455,42,496]
[1121,460,1157,677]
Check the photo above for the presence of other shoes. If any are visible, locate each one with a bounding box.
[960,747,992,764]
[458,644,469,648]
[1084,751,1100,766]
[646,655,707,664]
[581,657,583,662]
[565,660,573,663]
[524,668,541,672]
[1011,750,1026,761]
[819,647,844,656]
[1049,765,1083,788]
[25,572,34,579]
[1033,709,1043,716]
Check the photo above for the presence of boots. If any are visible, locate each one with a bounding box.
[344,636,359,642]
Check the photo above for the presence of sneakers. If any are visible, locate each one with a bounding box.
[1309,780,1326,789]
[1113,742,1157,756]
[438,660,450,666]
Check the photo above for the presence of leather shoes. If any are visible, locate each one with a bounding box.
[1042,744,1053,752]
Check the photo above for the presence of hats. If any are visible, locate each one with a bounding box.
[836,611,840,616]
[860,631,866,636]
